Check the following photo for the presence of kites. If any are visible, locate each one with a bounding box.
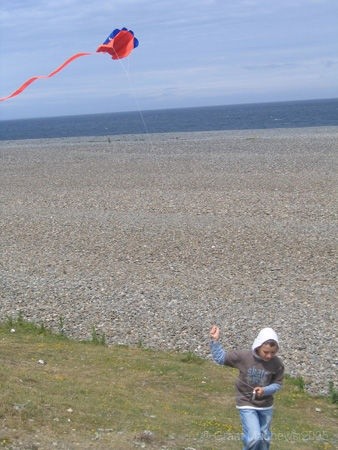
[0,26,139,103]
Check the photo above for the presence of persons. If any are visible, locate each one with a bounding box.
[209,325,286,450]
